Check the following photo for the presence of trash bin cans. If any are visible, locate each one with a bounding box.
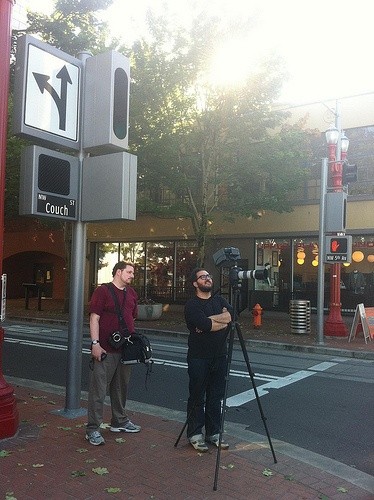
[290,299,311,334]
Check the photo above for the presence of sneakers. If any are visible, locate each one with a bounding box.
[110,419,141,433]
[85,430,106,446]
[190,438,208,453]
[205,434,229,448]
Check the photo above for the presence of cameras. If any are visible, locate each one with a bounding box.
[229,265,269,289]
[112,331,123,342]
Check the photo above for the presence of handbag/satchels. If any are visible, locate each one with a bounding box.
[122,333,152,362]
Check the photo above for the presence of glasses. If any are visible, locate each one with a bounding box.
[195,274,212,280]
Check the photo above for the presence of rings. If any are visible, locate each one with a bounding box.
[96,358,97,359]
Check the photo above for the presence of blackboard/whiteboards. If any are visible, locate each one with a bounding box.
[350,303,369,339]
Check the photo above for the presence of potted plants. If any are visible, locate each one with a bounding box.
[136,296,162,321]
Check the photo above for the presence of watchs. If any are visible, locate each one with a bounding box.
[91,340,99,345]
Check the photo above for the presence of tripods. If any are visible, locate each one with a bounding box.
[175,290,278,491]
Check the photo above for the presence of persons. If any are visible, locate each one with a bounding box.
[184,269,239,451]
[83,260,142,446]
[348,270,363,291]
[264,263,271,287]
